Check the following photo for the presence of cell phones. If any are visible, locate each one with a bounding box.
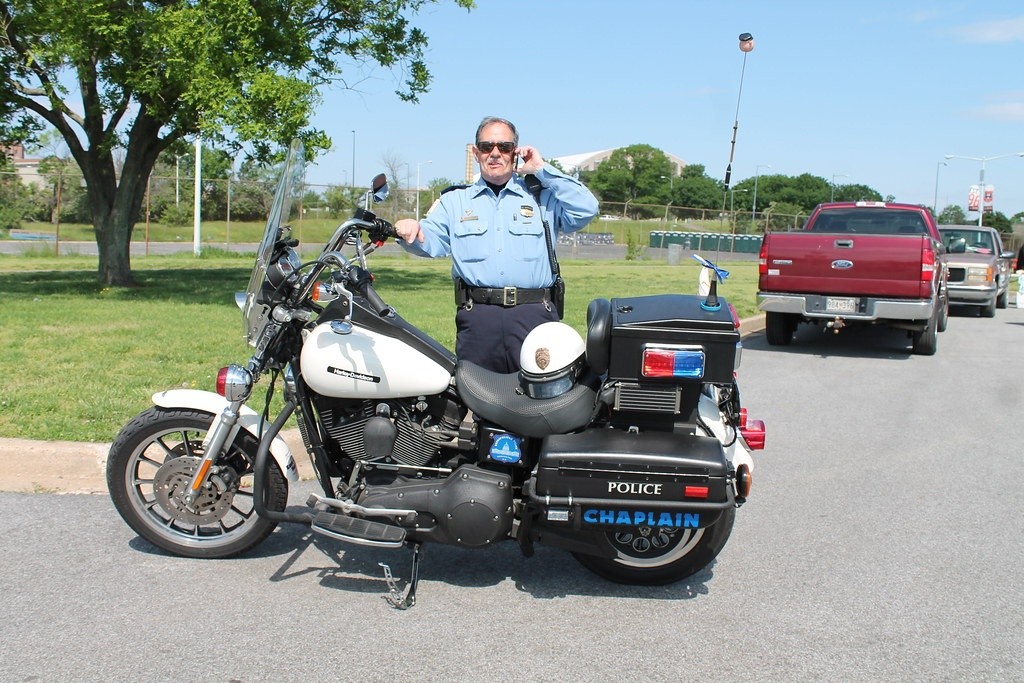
[513,156,518,170]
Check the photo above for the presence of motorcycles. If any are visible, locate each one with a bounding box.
[103,171,767,611]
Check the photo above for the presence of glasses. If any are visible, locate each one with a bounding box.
[477,141,515,153]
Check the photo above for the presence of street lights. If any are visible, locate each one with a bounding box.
[933,161,947,214]
[405,163,410,203]
[660,175,673,205]
[416,160,433,222]
[944,153,1024,242]
[752,164,770,220]
[831,173,849,203]
[730,189,748,233]
[352,130,356,193]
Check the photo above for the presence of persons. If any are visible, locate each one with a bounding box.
[393,116,600,375]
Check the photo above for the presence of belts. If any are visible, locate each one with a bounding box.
[467,288,552,306]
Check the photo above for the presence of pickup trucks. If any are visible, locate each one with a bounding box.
[754,200,967,356]
[934,224,1015,318]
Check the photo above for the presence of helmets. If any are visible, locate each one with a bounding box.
[519,322,587,398]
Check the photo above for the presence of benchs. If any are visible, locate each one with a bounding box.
[9,230,59,241]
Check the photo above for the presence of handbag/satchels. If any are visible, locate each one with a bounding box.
[551,276,564,319]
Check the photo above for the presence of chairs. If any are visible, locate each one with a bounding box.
[899,224,919,234]
[826,220,848,233]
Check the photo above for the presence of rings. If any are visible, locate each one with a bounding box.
[395,226,401,230]
